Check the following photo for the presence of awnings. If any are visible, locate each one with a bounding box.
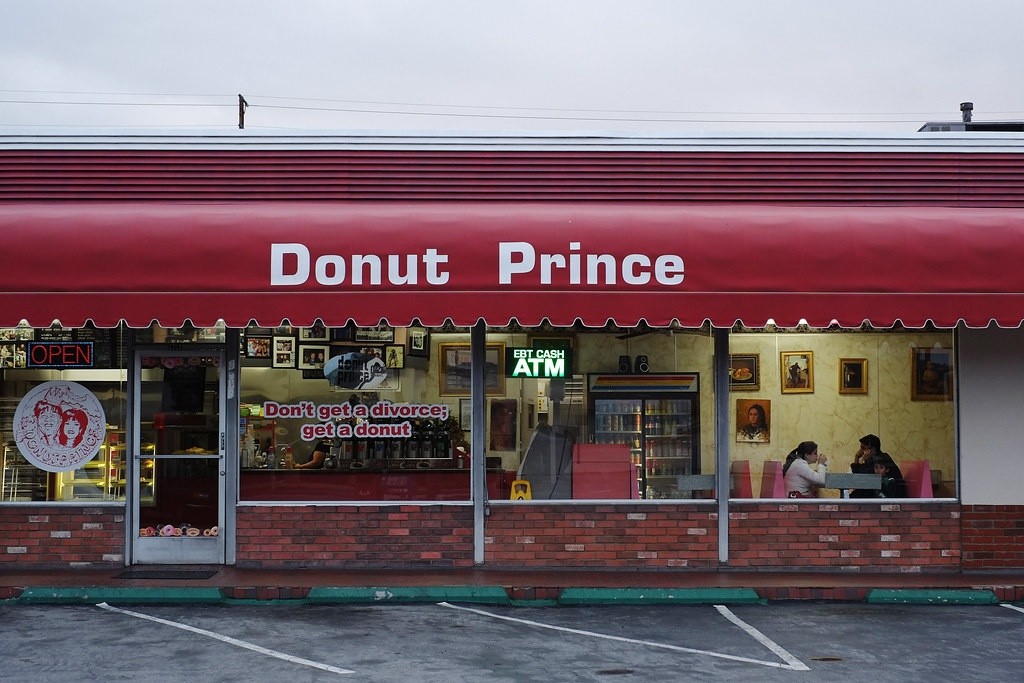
[0,203,1024,329]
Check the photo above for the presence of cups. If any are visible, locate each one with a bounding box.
[817,455,830,467]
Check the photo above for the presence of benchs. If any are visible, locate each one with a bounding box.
[760,462,785,499]
[731,460,753,498]
[900,460,932,498]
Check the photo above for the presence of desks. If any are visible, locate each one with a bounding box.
[630,464,639,499]
[678,474,734,491]
[815,473,882,498]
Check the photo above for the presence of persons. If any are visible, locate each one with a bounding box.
[736,404,769,440]
[247,326,422,367]
[850,434,905,498]
[0,330,34,367]
[782,441,827,498]
[295,422,332,468]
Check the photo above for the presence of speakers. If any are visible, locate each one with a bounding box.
[635,355,649,374]
[618,356,631,375]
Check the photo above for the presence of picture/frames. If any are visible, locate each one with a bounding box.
[406,327,430,357]
[459,399,471,432]
[272,336,296,369]
[438,342,505,397]
[839,358,868,394]
[780,351,814,394]
[729,353,760,391]
[384,344,405,369]
[910,347,953,401]
[298,344,330,370]
[245,337,272,358]
[245,320,272,335]
[298,322,331,342]
[355,320,395,343]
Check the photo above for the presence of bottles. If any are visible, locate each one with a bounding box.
[285,447,293,470]
[266,447,279,470]
[644,439,692,499]
[644,415,692,436]
[341,413,454,462]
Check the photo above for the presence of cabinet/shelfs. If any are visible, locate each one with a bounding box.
[2,429,155,502]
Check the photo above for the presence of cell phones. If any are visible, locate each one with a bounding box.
[871,448,876,455]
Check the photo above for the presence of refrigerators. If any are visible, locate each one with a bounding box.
[583,371,703,499]
